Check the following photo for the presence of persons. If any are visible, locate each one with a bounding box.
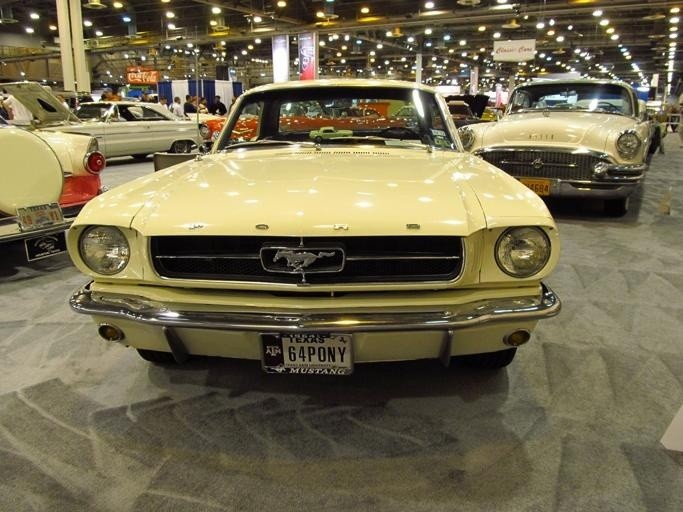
[56,93,69,112]
[659,101,683,149]
[81,91,236,122]
[0,96,11,125]
[3,91,33,122]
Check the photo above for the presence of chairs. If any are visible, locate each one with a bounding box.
[377,127,420,140]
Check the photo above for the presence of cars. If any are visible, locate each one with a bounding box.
[455,79,657,215]
[200,105,417,145]
[66,78,562,376]
[0,81,203,261]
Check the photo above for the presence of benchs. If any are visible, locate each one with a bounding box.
[277,130,381,141]
